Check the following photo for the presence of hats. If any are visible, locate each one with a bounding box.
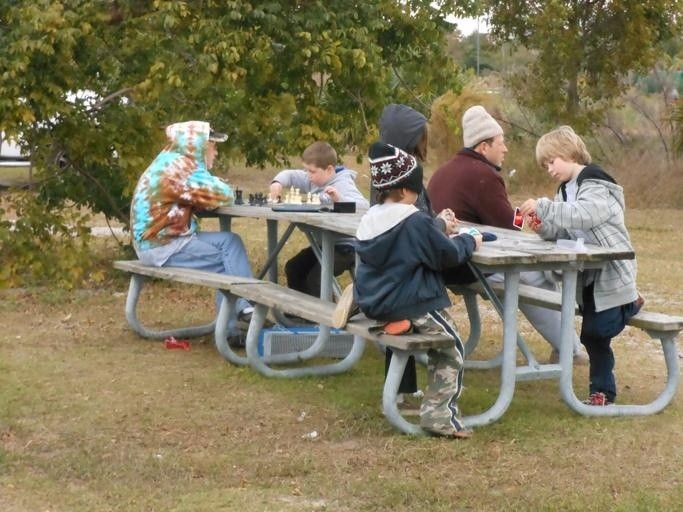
[209,127,229,143]
[461,105,503,149]
[368,141,423,193]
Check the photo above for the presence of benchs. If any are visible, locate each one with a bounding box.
[224,278,457,436]
[111,253,270,370]
[442,273,682,419]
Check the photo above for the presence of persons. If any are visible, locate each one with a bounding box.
[427,105,590,364]
[518,126,645,406]
[355,142,483,439]
[269,140,370,324]
[378,103,436,217]
[130,120,275,348]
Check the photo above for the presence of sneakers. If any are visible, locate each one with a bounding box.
[218,335,247,350]
[241,305,277,329]
[550,350,590,365]
[581,392,615,406]
[634,290,645,309]
[424,424,473,438]
[397,389,425,404]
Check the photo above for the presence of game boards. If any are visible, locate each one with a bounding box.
[232,184,281,206]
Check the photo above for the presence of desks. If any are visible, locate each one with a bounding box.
[286,208,682,437]
[124,193,360,379]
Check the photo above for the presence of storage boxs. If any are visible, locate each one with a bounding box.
[254,321,353,358]
